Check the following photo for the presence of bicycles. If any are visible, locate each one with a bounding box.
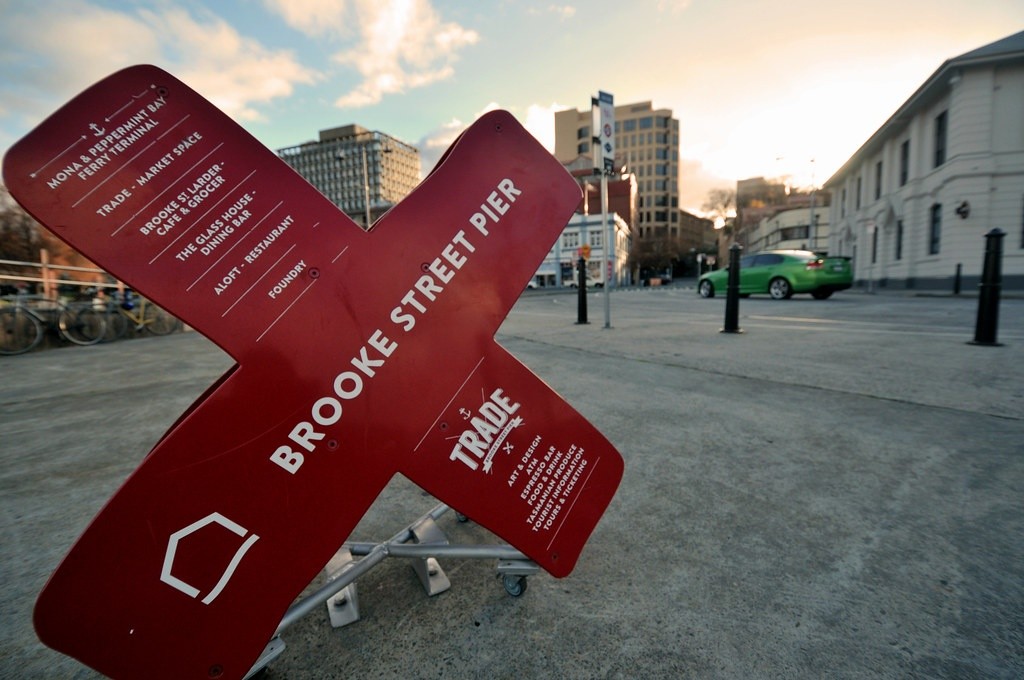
[0,284,183,356]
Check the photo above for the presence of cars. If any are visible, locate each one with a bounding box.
[696,249,854,301]
[525,281,537,290]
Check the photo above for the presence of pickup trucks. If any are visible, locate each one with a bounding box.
[564,273,604,288]
